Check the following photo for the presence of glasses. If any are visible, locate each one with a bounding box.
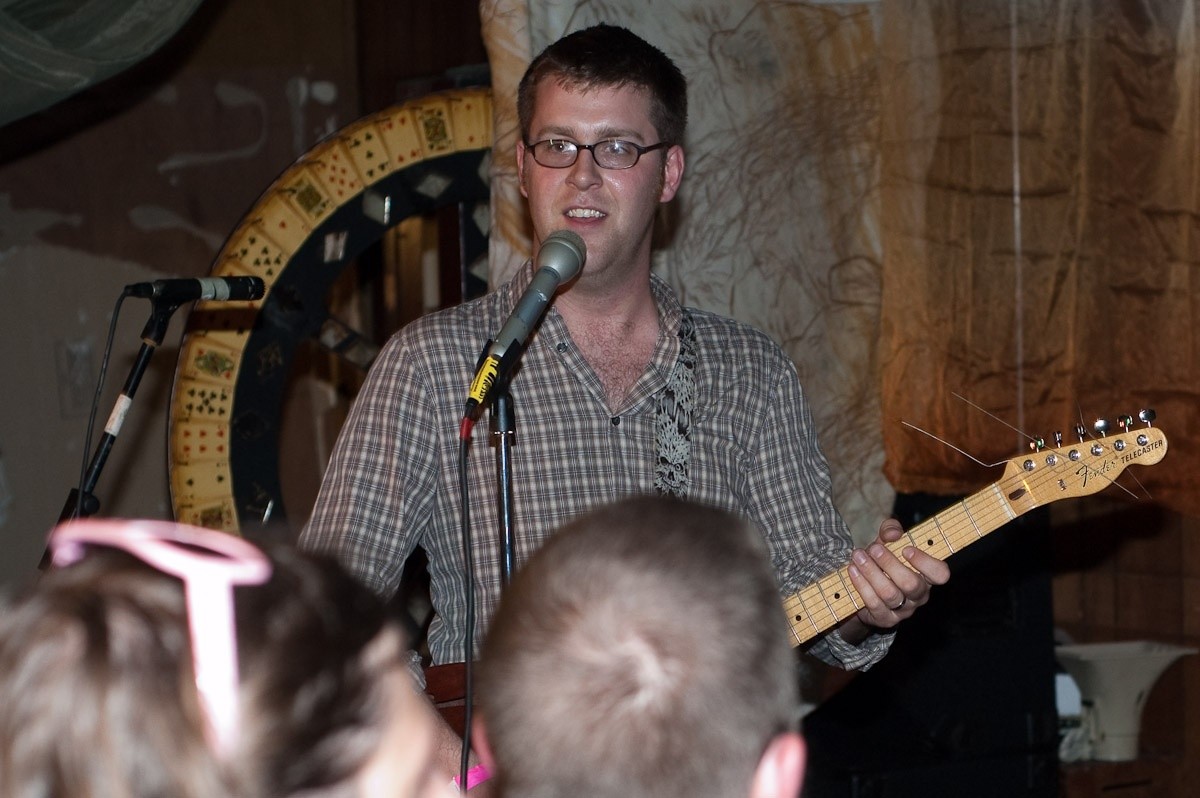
[522,138,672,169]
[47,519,269,751]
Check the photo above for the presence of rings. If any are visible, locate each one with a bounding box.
[889,594,906,610]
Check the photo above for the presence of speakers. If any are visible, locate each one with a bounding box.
[789,490,1061,798]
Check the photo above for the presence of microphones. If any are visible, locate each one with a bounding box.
[468,230,587,412]
[124,275,265,301]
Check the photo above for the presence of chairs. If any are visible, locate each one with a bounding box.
[794,494,1063,798]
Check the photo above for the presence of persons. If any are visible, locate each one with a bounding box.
[0,520,484,798]
[471,496,804,798]
[294,17,950,797]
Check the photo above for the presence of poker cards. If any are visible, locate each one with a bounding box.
[167,79,495,538]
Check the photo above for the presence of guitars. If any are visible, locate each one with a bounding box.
[419,408,1169,785]
